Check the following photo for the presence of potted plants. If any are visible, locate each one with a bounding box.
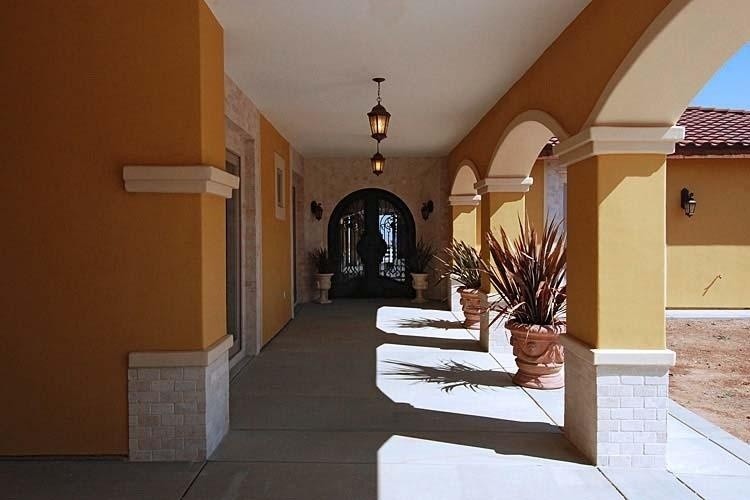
[404,237,438,305]
[426,238,481,329]
[309,244,339,304]
[479,209,570,391]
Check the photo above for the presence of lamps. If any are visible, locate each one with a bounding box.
[370,140,385,176]
[681,187,697,218]
[367,78,391,143]
[421,200,434,221]
[310,200,323,221]
